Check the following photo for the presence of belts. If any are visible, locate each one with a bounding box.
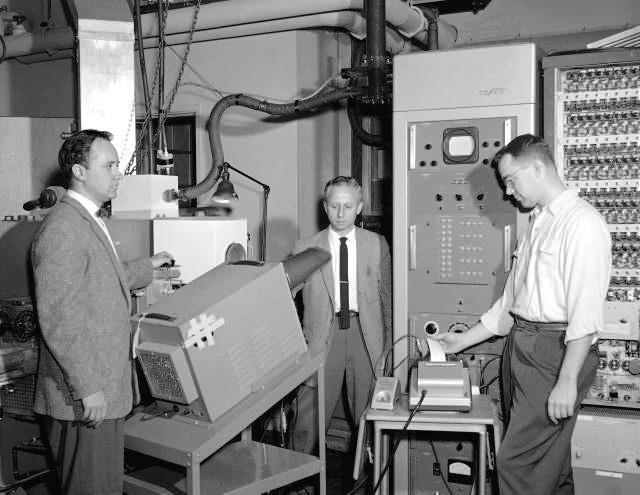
[516,319,568,330]
[334,311,359,318]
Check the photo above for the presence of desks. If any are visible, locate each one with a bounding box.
[353,395,501,495]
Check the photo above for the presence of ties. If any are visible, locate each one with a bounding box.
[340,237,350,329]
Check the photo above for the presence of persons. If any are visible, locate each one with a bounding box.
[288,176,393,456]
[430,134,614,495]
[32,130,174,495]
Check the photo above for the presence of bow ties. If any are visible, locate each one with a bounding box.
[96,208,108,217]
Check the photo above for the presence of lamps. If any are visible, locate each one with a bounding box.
[210,161,270,260]
